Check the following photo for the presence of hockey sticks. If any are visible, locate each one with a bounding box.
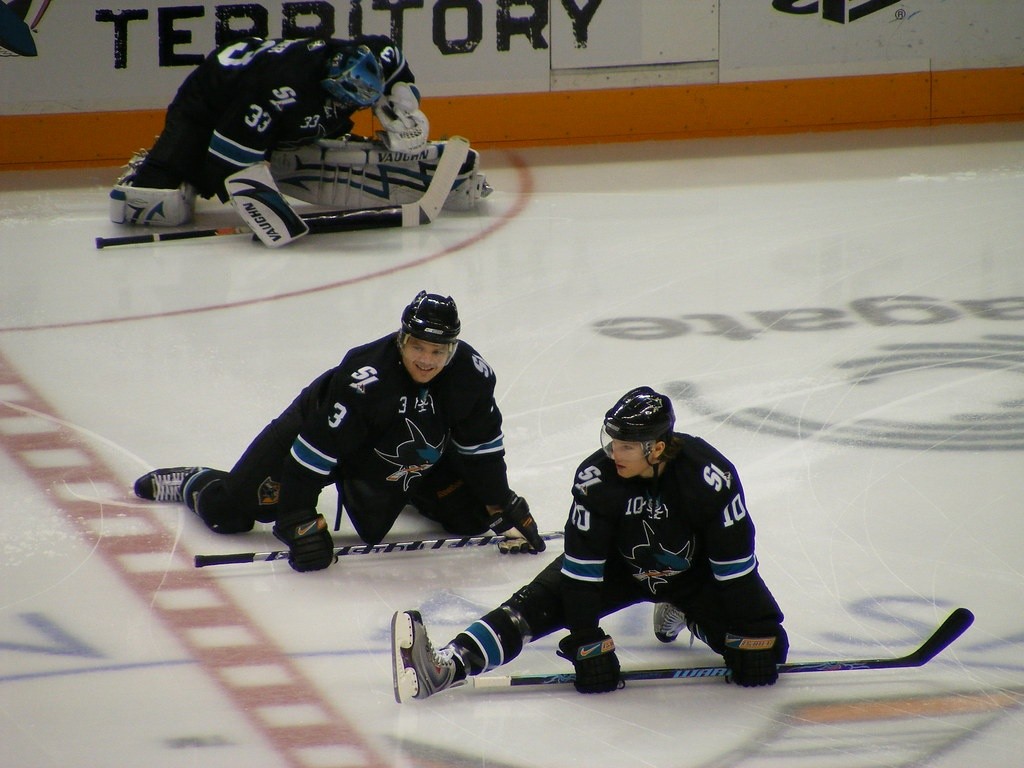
[95,134,469,248]
[193,531,565,569]
[472,607,975,689]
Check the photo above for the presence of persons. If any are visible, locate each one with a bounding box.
[133,289,545,572]
[391,386,789,703]
[109,33,493,252]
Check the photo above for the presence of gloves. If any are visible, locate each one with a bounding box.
[273,508,334,573]
[724,628,778,687]
[557,627,621,694]
[486,488,547,554]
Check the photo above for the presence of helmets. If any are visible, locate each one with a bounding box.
[401,290,461,345]
[322,44,385,107]
[604,386,675,441]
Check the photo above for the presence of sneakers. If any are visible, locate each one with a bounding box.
[390,610,468,704]
[133,466,208,502]
[654,602,687,643]
